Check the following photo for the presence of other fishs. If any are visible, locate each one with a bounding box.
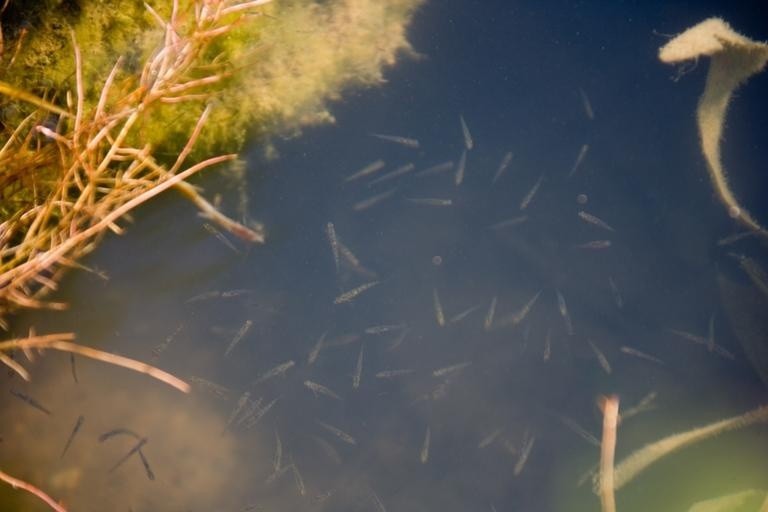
[12,118,768,512]
